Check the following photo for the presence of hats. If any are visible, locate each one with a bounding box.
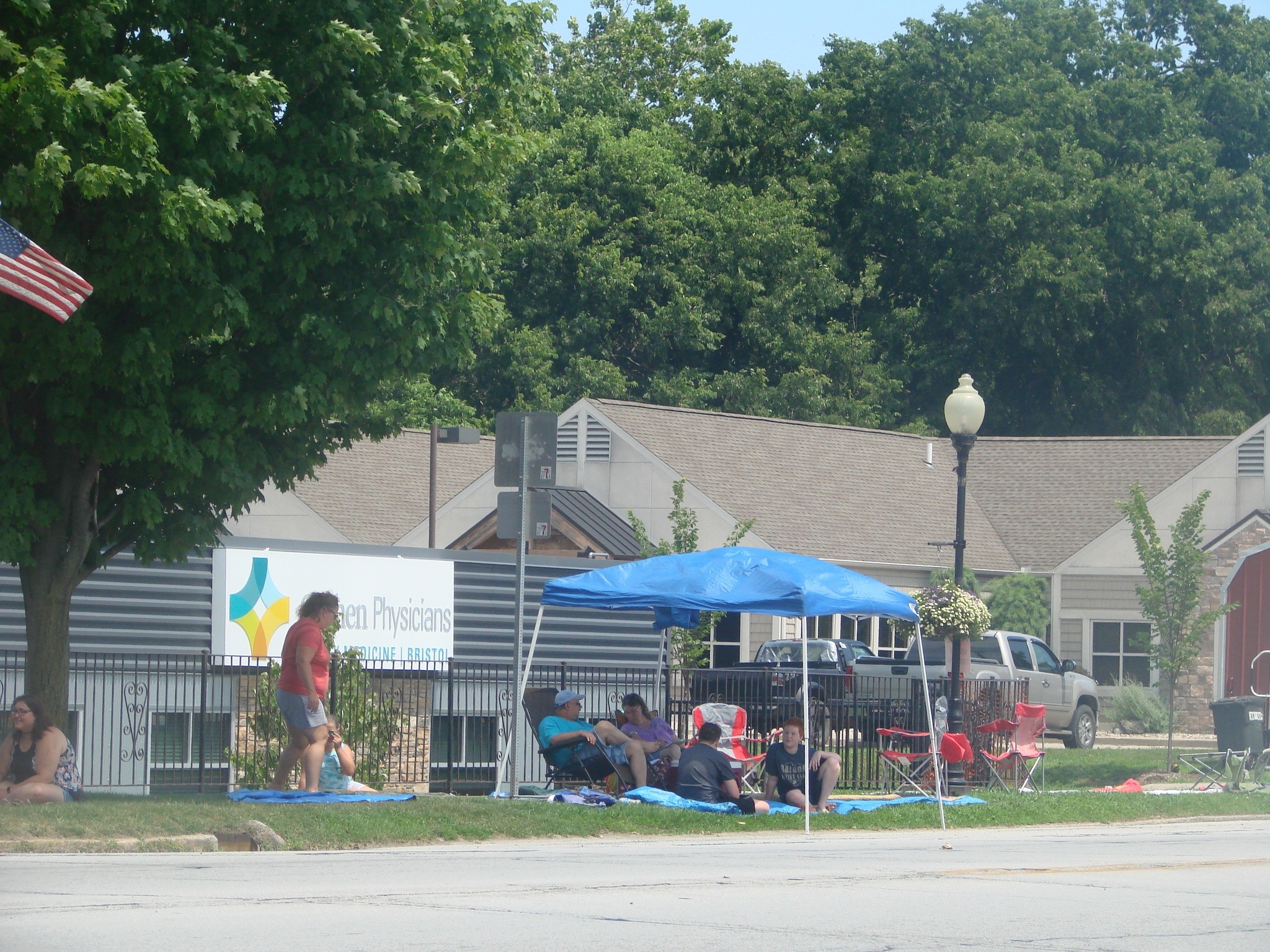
[554,689,586,708]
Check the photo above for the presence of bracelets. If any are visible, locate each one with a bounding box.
[630,731,637,736]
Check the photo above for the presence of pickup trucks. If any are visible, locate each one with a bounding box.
[690,638,876,746]
[844,628,1100,750]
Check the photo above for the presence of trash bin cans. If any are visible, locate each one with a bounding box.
[1209,696,1264,771]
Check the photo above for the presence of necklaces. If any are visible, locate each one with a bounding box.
[21,732,31,734]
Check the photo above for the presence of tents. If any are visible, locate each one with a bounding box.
[493,547,945,830]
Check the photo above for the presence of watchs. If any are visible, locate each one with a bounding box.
[7,787,11,794]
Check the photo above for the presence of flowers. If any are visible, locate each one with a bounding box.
[888,580,990,642]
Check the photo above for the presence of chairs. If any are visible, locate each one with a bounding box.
[688,702,784,796]
[978,702,1046,799]
[875,695,949,799]
[1177,747,1270,794]
[521,687,683,796]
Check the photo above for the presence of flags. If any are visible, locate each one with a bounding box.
[0,219,94,323]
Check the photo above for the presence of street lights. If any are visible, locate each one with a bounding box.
[931,372,986,798]
[428,423,480,548]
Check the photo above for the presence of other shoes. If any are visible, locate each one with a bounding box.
[654,772,676,793]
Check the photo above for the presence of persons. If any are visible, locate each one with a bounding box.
[765,717,841,812]
[676,723,770,815]
[267,592,339,792]
[538,690,685,789]
[0,695,83,804]
[317,714,378,793]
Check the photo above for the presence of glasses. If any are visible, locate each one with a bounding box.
[328,730,337,738]
[10,707,32,716]
[327,608,339,620]
[568,702,580,706]
[623,705,641,717]
